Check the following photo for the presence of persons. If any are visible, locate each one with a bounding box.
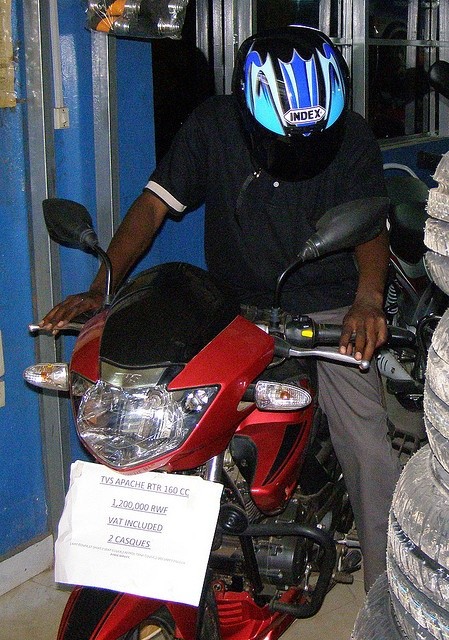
[39,25,403,592]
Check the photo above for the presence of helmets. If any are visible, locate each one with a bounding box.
[231,24,349,182]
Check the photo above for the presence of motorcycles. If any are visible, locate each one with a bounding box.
[374,60,449,412]
[24,186,421,638]
